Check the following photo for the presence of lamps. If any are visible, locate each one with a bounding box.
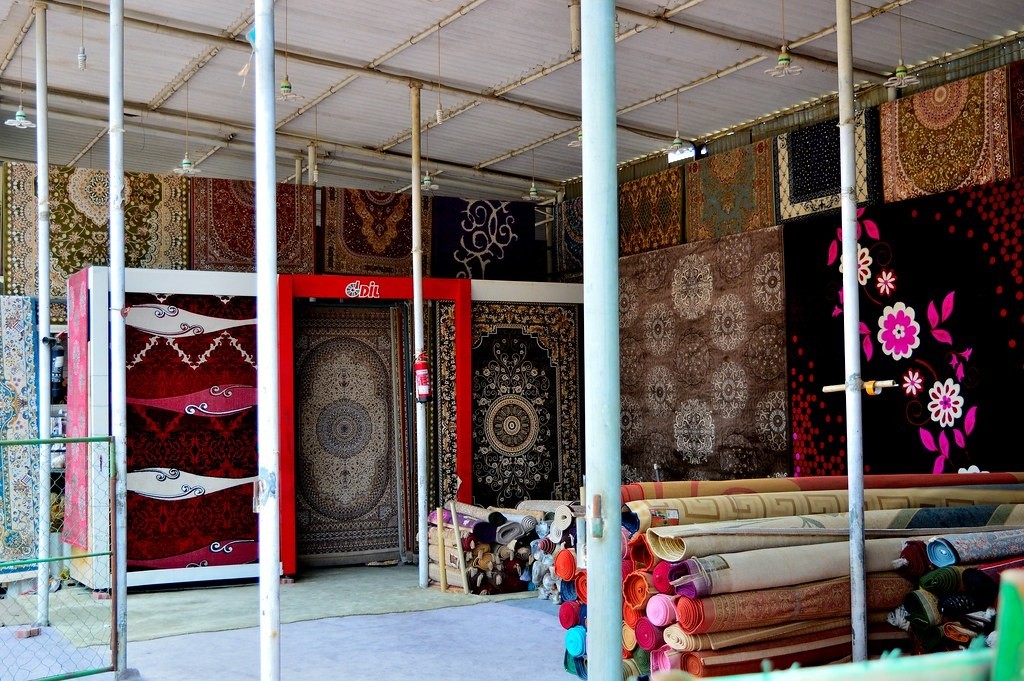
[174,82,201,178]
[274,0,306,102]
[421,129,439,192]
[669,92,695,162]
[764,0,804,79]
[882,5,920,88]
[522,149,545,202]
[568,130,582,148]
[4,45,36,129]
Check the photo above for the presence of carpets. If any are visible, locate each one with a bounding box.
[0,28,1024,681]
